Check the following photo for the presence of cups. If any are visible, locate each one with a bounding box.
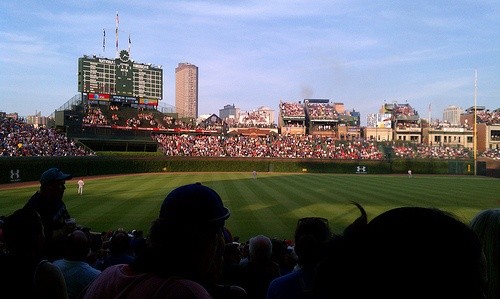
[64,219,75,236]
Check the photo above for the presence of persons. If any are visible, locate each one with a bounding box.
[77,177,85,195]
[82,103,500,160]
[408,170,412,178]
[80,182,232,299]
[0,114,97,157]
[0,167,500,299]
[251,170,256,178]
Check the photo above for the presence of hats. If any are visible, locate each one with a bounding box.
[159,182,230,225]
[41,167,74,182]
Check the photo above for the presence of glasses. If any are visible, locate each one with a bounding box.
[297,216,330,226]
